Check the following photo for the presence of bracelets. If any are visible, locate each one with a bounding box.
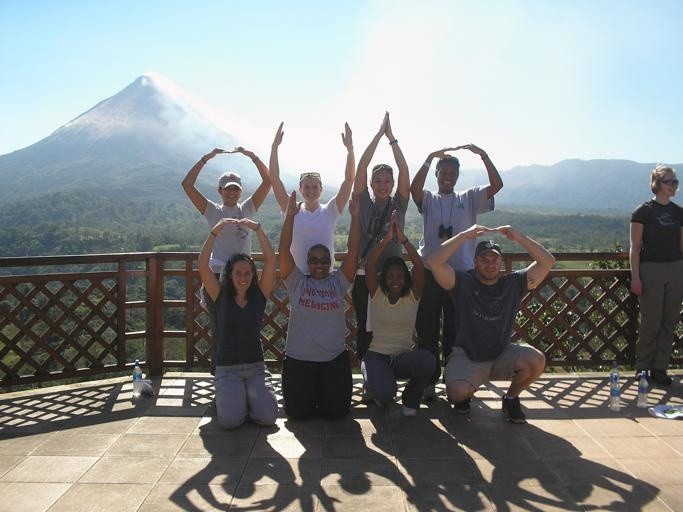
[390,139,398,144]
[210,231,216,237]
[399,238,409,243]
[422,161,431,167]
[483,154,490,159]
[252,156,259,164]
[201,157,207,165]
[252,222,262,232]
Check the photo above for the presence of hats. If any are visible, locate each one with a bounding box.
[219,171,243,190]
[475,240,502,257]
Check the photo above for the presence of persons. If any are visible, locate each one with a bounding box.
[361,208,436,416]
[267,120,355,278]
[199,217,279,429]
[277,191,360,421]
[184,148,273,376]
[410,144,503,401]
[629,166,683,384]
[351,110,410,368]
[426,223,556,423]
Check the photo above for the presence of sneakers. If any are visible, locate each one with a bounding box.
[636,370,648,381]
[650,368,672,385]
[361,381,526,424]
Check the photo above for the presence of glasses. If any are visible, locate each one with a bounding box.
[301,172,320,180]
[662,179,679,185]
[308,256,330,264]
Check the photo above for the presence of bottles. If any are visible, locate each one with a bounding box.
[132,359,143,397]
[608,360,649,412]
